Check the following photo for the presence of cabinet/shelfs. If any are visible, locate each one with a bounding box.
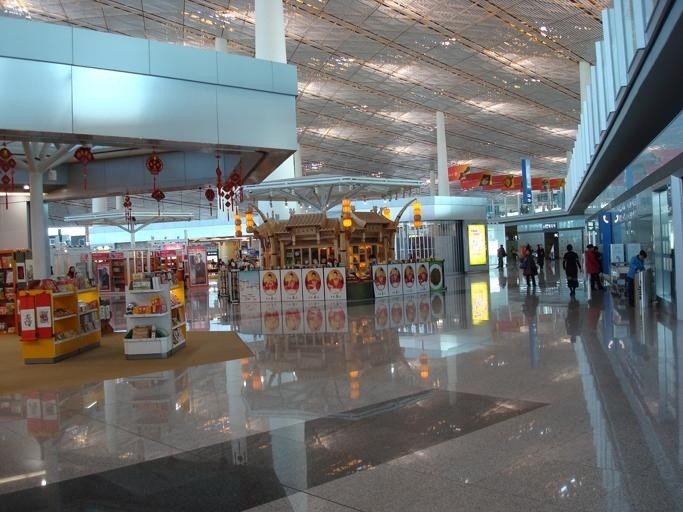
[92,250,184,292]
[0,249,24,336]
[21,287,99,364]
[124,282,186,361]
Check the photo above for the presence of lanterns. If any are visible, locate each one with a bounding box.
[245,214,253,221]
[342,219,351,227]
[412,209,421,215]
[412,201,421,209]
[245,207,252,214]
[233,214,240,220]
[341,207,351,213]
[341,213,351,219]
[234,226,241,231]
[413,215,421,221]
[234,231,242,237]
[383,208,389,215]
[234,220,241,226]
[245,221,253,226]
[341,199,351,207]
[245,226,253,233]
[413,221,421,228]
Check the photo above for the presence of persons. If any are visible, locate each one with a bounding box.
[66,266,74,278]
[375,269,386,286]
[50,266,53,275]
[262,273,277,294]
[592,245,601,283]
[625,249,646,307]
[405,267,413,284]
[305,272,319,290]
[518,248,537,287]
[534,243,544,269]
[207,254,260,271]
[525,244,532,259]
[509,246,518,271]
[584,244,604,291]
[327,271,343,291]
[417,264,427,285]
[389,268,400,285]
[562,244,581,296]
[283,273,299,291]
[495,244,506,270]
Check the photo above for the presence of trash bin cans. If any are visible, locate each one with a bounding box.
[634,268,657,306]
